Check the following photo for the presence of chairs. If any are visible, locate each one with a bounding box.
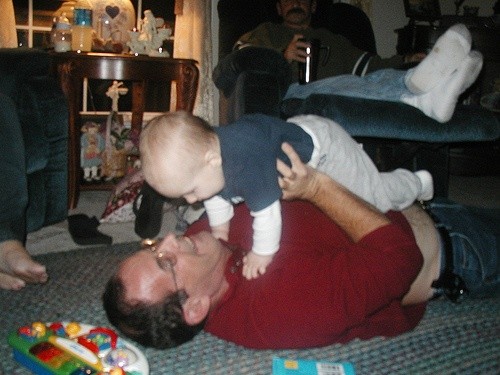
[217,0,500,200]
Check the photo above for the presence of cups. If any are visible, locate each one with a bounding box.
[53,23,71,53]
[298,36,331,85]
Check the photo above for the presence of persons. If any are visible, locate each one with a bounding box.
[234,1,484,123]
[103,193,500,350]
[0,92,49,292]
[137,111,447,280]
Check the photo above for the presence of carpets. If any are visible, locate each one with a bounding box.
[0,236,500,375]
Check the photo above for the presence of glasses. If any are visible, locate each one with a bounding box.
[141,238,180,295]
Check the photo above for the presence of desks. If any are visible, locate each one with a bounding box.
[49,50,199,211]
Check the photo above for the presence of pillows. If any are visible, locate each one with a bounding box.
[98,168,174,224]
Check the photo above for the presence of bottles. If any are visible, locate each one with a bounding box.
[71,0,93,52]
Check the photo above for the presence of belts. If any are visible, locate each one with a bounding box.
[416,201,455,296]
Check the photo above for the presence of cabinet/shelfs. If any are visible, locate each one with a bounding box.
[395,25,500,167]
